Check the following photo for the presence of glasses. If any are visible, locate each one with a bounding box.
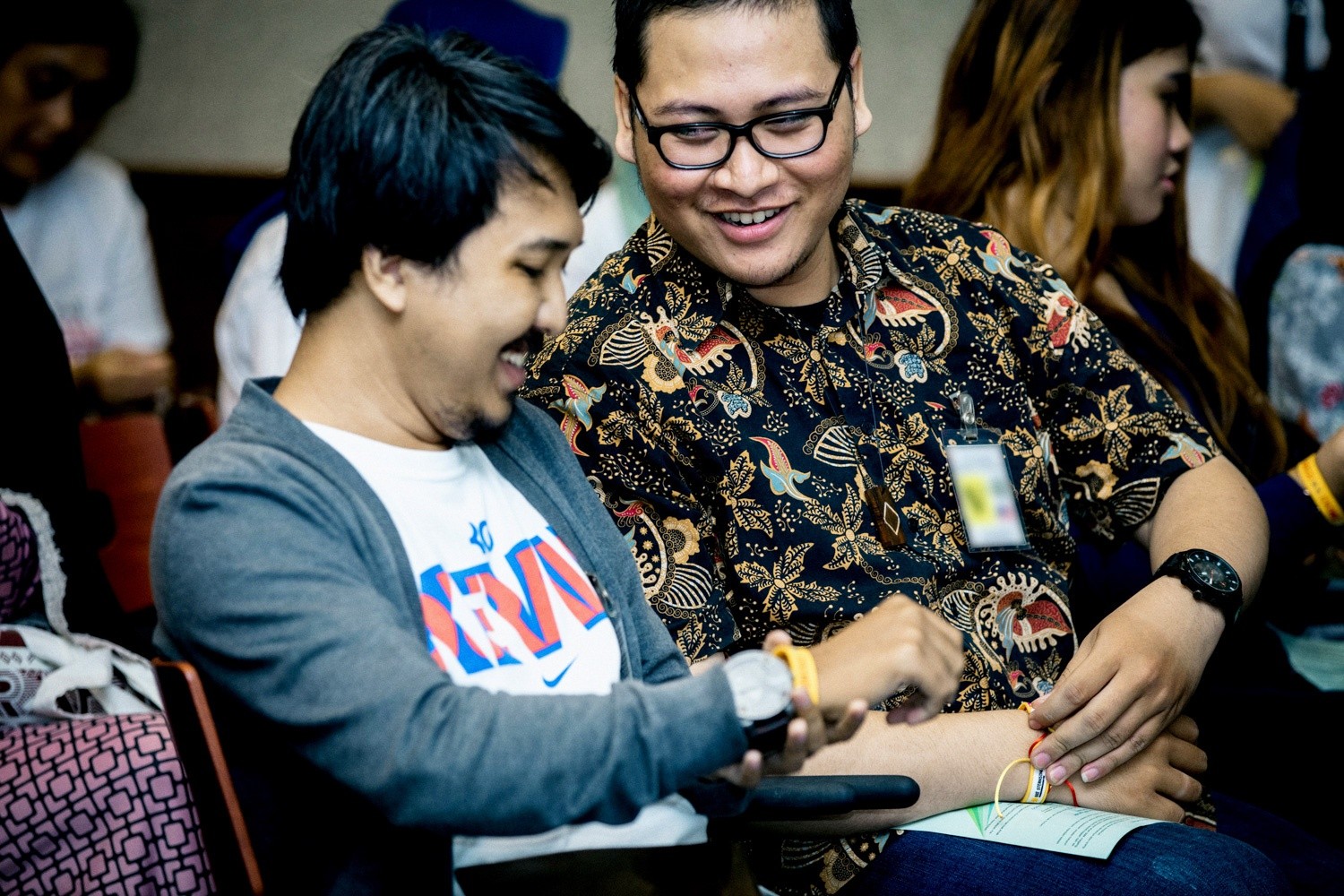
[627,62,851,170]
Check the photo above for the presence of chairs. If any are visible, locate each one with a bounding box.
[153,657,855,896]
[73,350,215,611]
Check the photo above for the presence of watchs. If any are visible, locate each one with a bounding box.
[1150,547,1246,630]
[723,649,796,771]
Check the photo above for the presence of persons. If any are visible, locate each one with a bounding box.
[900,0,1343,860]
[210,0,625,425]
[153,16,967,895]
[0,0,216,655]
[516,0,1287,896]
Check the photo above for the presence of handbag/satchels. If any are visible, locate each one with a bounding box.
[0,622,219,896]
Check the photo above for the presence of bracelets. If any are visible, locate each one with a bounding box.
[994,700,1082,816]
[1295,451,1344,524]
[772,643,822,706]
